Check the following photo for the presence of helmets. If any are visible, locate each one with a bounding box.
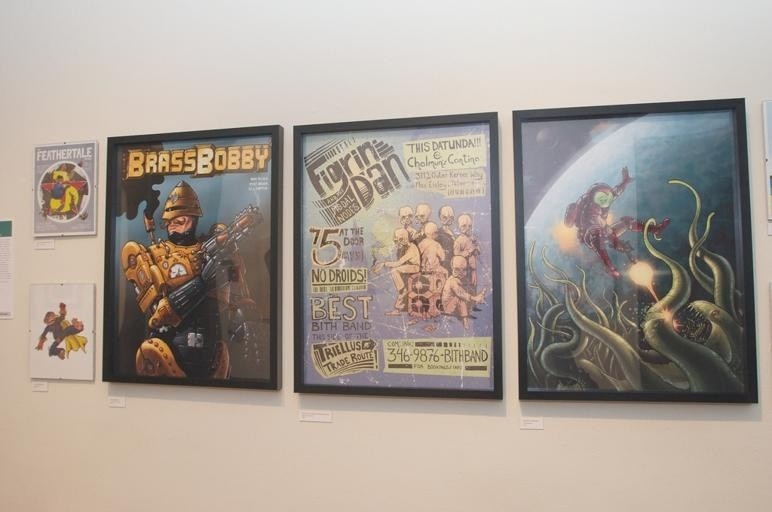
[161,180,202,220]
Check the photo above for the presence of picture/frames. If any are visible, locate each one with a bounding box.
[510,98,761,404]
[30,140,98,236]
[292,111,503,399]
[103,124,284,391]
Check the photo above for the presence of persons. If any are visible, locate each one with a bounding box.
[371,203,485,331]
[35,303,84,360]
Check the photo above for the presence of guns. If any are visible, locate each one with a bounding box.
[202,206,263,290]
